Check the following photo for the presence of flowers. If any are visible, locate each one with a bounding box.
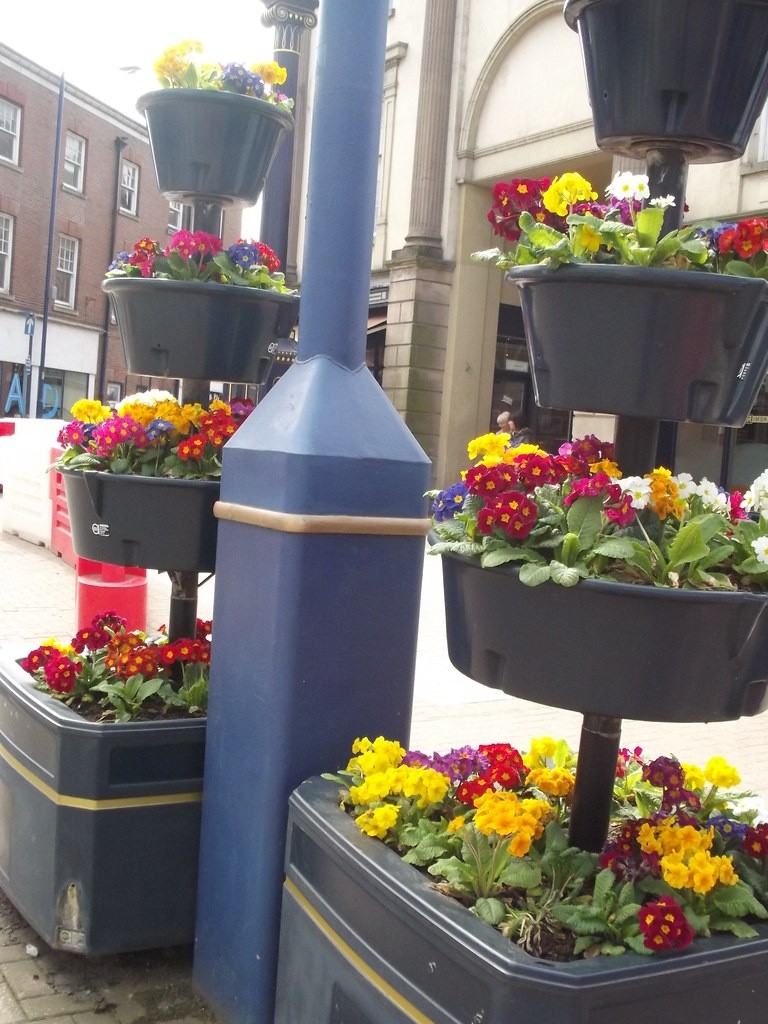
[471,171,768,278]
[24,608,213,724]
[38,388,256,481]
[155,37,294,114]
[339,732,768,962]
[103,227,300,295]
[428,432,768,591]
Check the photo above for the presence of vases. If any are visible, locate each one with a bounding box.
[53,464,220,575]
[504,262,768,429]
[563,0,768,166]
[0,640,207,963]
[100,277,300,381]
[426,532,768,723]
[271,776,768,1024]
[134,88,296,211]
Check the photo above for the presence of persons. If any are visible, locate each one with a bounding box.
[496,411,512,435]
[500,410,537,453]
